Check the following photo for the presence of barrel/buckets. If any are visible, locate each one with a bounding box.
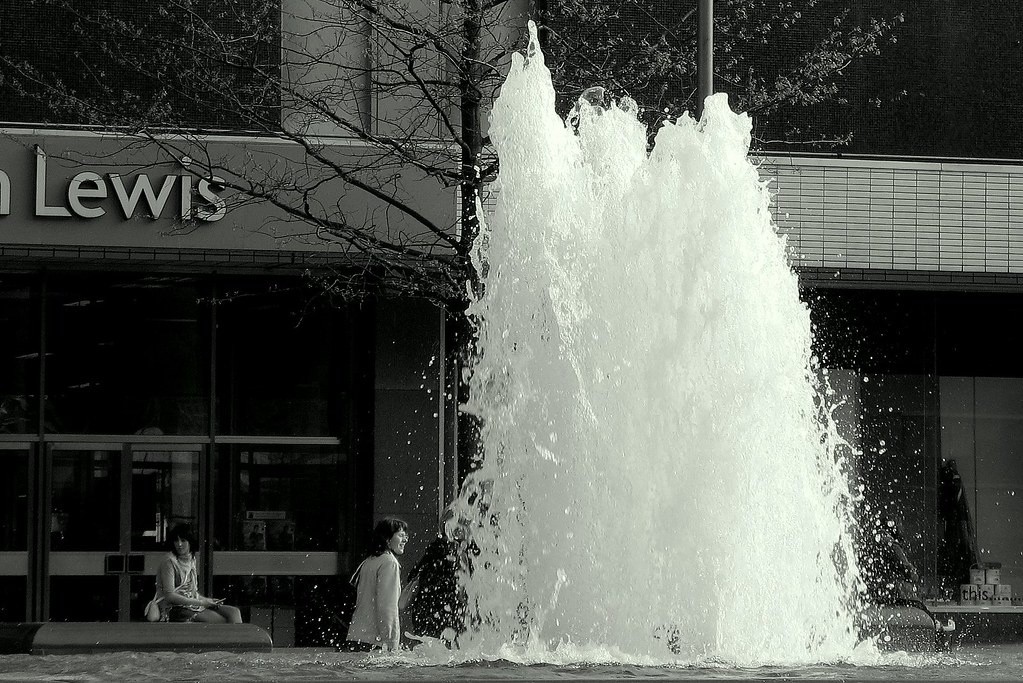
[995,584,1011,605]
[960,584,977,606]
[978,585,994,607]
[970,563,985,585]
[982,562,1001,584]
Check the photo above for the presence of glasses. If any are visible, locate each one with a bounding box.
[392,534,409,539]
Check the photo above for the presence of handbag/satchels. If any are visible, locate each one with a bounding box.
[145,600,160,621]
[326,558,367,629]
[399,574,421,611]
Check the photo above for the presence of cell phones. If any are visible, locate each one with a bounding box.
[214,598,226,604]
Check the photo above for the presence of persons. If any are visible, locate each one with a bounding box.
[408,504,469,650]
[860,513,936,624]
[345,517,410,652]
[156,523,242,623]
